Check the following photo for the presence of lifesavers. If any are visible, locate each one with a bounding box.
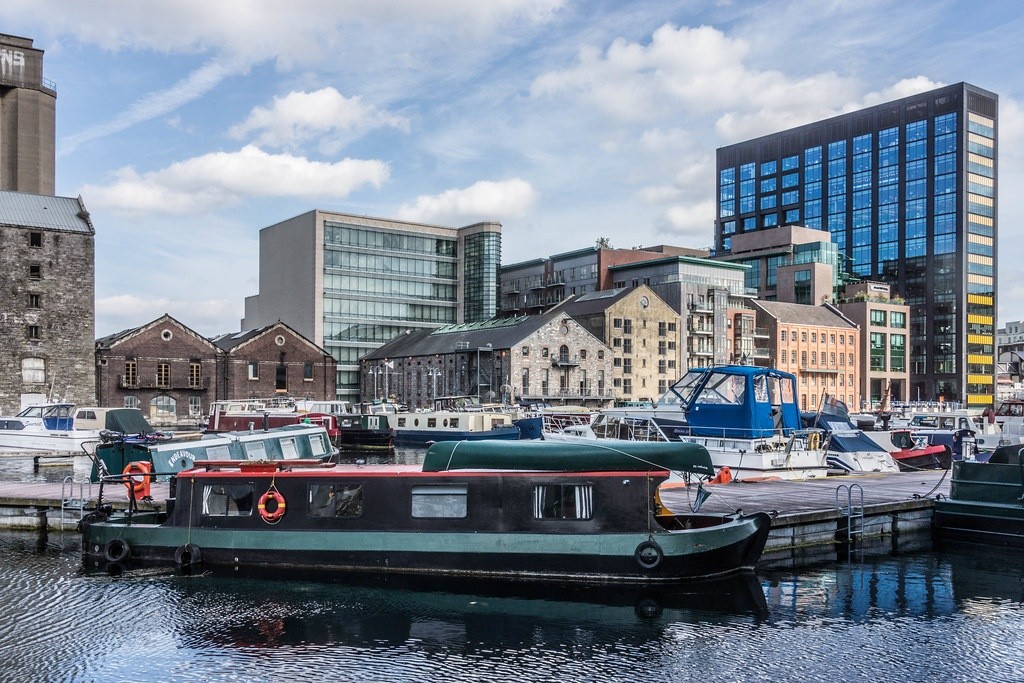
[258,491,285,521]
[808,433,819,450]
[634,540,661,569]
[105,539,129,564]
[174,544,202,563]
[123,461,148,491]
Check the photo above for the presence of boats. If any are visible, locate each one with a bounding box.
[189,362,1024,559]
[73,407,204,445]
[90,433,341,481]
[78,435,772,618]
[0,401,161,457]
